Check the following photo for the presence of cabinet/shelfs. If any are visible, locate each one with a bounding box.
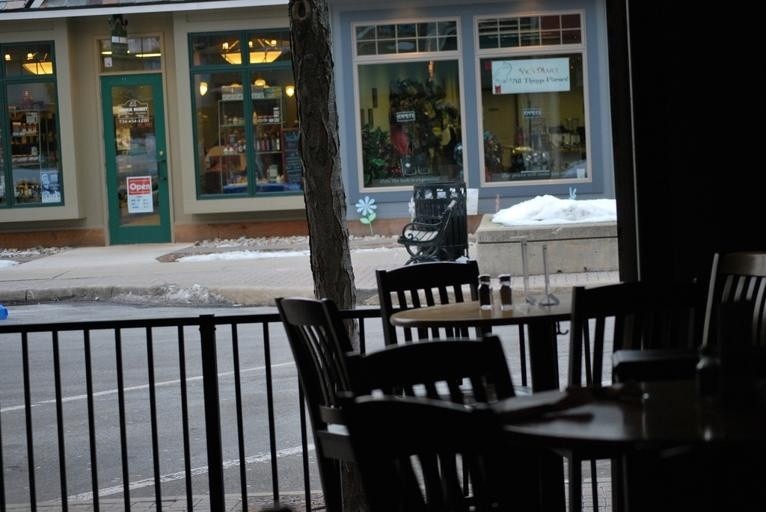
[216,97,286,193]
[6,103,56,205]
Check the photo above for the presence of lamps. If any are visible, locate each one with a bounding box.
[106,14,129,58]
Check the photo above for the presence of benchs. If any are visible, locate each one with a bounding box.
[397,199,461,267]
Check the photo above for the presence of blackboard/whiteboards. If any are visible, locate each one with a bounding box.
[281,128,302,184]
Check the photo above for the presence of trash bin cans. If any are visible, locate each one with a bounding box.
[412,182,469,258]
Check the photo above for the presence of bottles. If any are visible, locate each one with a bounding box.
[224,130,281,152]
[252,112,257,126]
[478,274,494,310]
[499,274,515,311]
